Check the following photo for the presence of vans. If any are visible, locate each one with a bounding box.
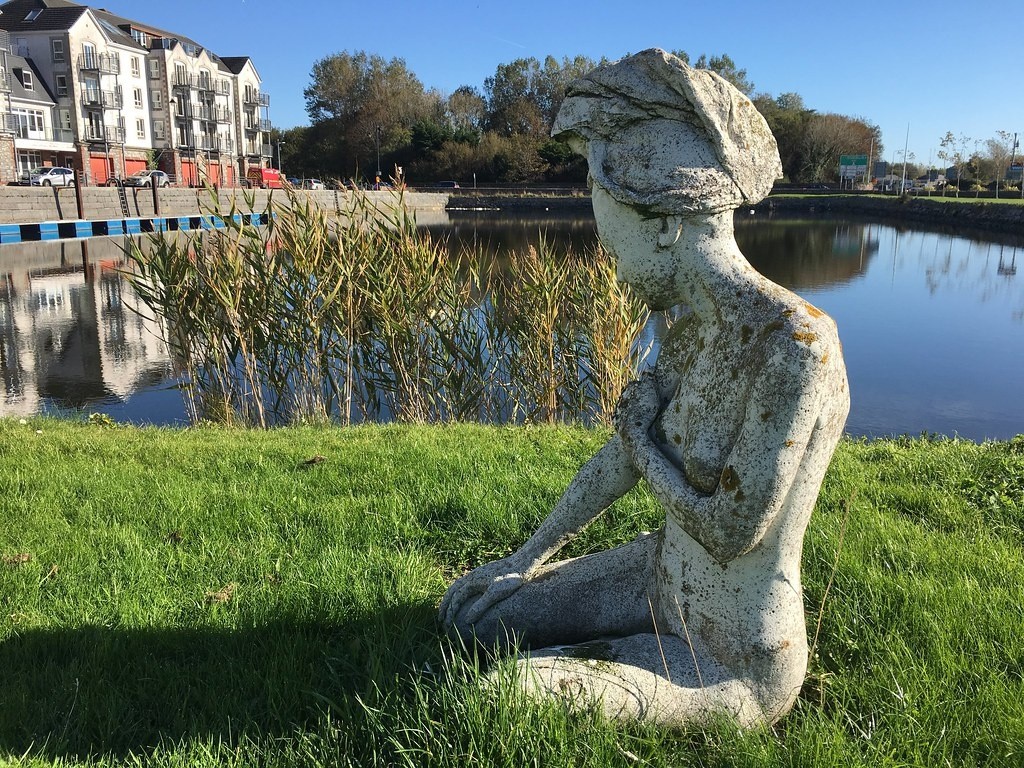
[247,166,287,189]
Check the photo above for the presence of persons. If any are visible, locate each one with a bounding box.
[433,49,851,734]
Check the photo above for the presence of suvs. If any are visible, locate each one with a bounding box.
[125,169,171,188]
[18,165,76,187]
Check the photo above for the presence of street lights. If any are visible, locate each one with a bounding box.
[277,139,286,172]
[169,87,193,188]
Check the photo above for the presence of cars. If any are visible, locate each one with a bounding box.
[433,180,460,187]
[371,182,392,190]
[287,178,367,190]
